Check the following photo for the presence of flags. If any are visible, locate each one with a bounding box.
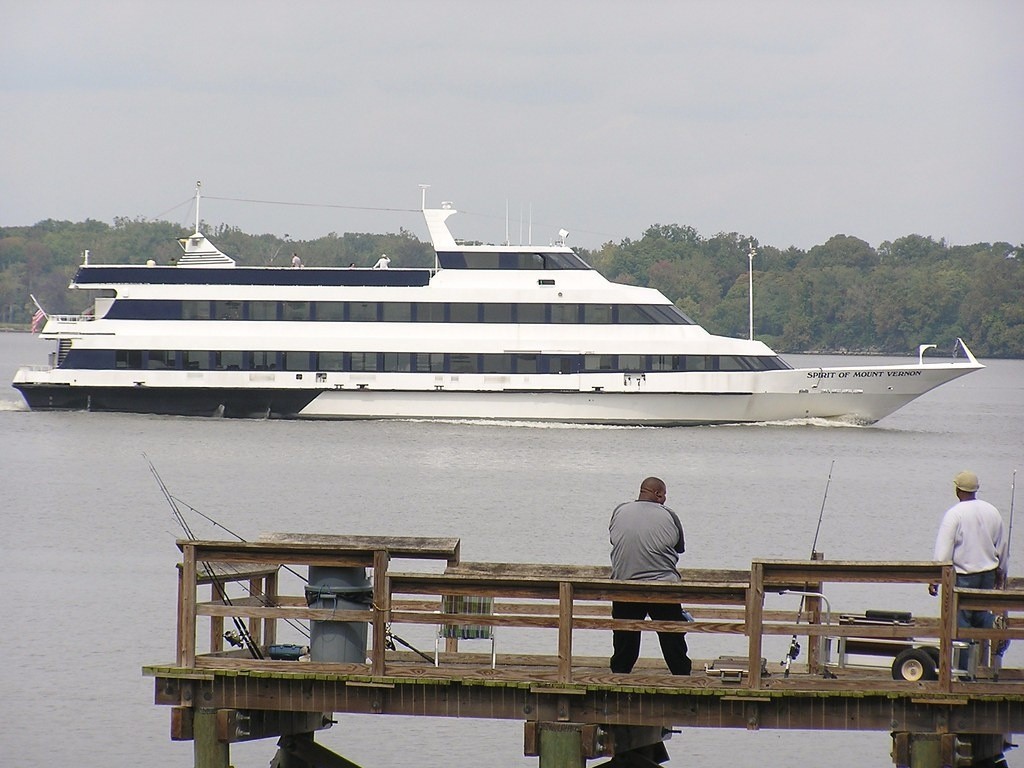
[31,308,44,335]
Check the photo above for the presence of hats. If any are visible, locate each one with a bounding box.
[953,472,979,492]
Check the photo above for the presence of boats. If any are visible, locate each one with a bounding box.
[10,201,988,428]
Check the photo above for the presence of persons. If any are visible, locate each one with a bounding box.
[608,476,692,675]
[927,471,1003,681]
[372,253,391,268]
[291,252,301,267]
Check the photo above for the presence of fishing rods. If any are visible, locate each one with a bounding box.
[779,459,837,678]
[992,469,1020,684]
[138,450,442,666]
